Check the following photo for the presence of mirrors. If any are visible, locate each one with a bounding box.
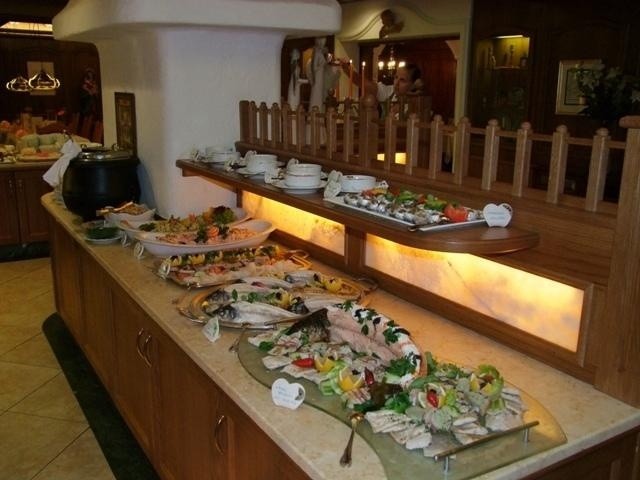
[0,34,101,148]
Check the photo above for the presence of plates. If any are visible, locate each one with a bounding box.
[87,206,378,327]
[238,167,282,179]
[271,181,329,193]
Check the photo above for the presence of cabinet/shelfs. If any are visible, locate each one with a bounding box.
[48,214,113,399]
[470,25,537,132]
[0,169,53,248]
[210,382,313,479]
[113,280,210,480]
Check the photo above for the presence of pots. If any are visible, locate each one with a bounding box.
[61,150,141,213]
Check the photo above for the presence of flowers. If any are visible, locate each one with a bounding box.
[567,64,640,125]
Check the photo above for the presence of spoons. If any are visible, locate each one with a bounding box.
[338,409,363,469]
[229,322,251,352]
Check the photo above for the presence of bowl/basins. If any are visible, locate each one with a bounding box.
[246,154,278,171]
[285,163,321,185]
[338,175,376,192]
[209,152,240,161]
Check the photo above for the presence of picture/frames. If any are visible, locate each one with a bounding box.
[555,59,606,118]
[113,91,138,151]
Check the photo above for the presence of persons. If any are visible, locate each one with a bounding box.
[329,59,424,124]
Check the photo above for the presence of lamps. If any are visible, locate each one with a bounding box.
[28,32,60,91]
[5,36,28,92]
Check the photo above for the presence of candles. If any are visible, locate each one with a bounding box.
[361,59,366,101]
[335,62,341,100]
[348,59,354,99]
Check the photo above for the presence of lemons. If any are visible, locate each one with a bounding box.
[338,365,365,392]
[313,351,338,373]
